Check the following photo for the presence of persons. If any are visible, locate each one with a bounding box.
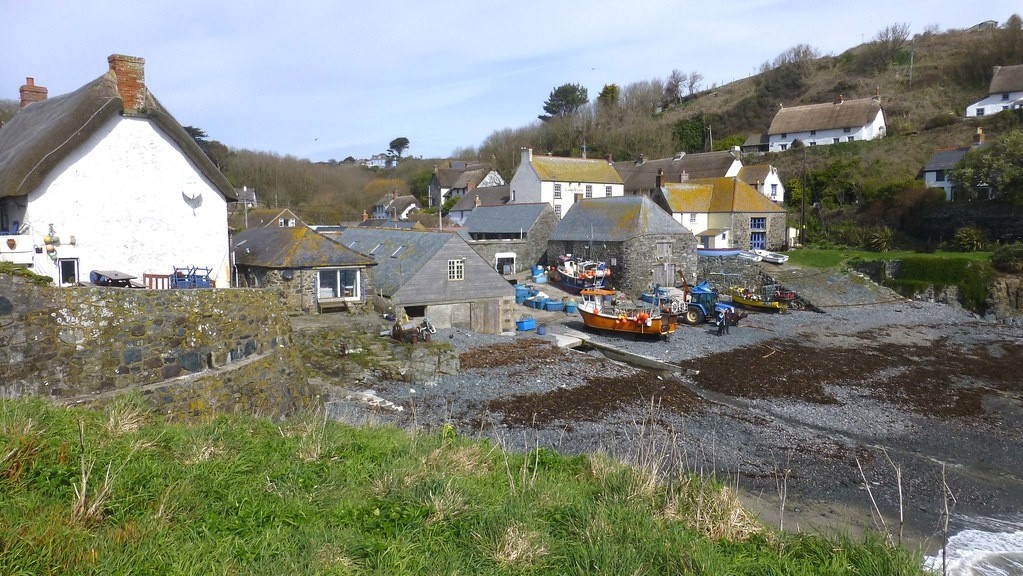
[717,310,730,337]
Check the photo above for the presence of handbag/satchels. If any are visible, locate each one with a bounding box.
[716,321,720,325]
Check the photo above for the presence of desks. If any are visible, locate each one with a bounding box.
[94,271,138,287]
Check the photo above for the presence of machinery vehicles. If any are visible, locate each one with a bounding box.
[684,285,738,326]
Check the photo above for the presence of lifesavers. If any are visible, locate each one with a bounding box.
[679,300,685,311]
[671,302,678,313]
[774,291,780,296]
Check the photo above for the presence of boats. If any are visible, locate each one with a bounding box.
[742,253,762,262]
[554,255,606,291]
[748,250,789,265]
[730,280,795,313]
[642,284,684,307]
[577,286,677,335]
[697,246,741,255]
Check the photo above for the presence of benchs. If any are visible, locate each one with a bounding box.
[128,280,149,287]
[78,281,98,287]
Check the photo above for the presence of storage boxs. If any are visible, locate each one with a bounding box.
[513,266,577,335]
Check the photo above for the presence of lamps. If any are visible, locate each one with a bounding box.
[283,270,293,279]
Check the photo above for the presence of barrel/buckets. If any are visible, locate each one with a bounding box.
[537,326,546,335]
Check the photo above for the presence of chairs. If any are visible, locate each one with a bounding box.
[169,265,213,287]
[0,220,19,235]
